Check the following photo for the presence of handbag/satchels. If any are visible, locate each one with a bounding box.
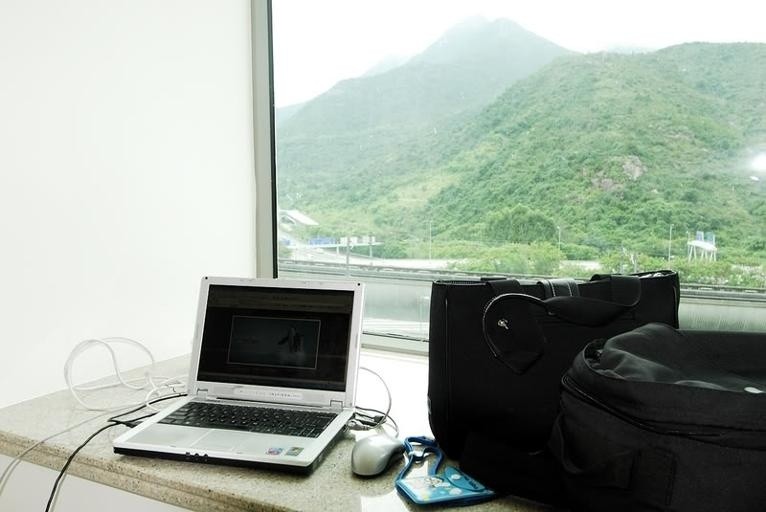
[548,321,765,511]
[428,269,680,491]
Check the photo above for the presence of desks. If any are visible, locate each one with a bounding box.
[0,347,551,512]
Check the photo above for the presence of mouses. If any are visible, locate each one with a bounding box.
[350,431,407,481]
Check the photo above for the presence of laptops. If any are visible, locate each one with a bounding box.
[111,274,368,476]
